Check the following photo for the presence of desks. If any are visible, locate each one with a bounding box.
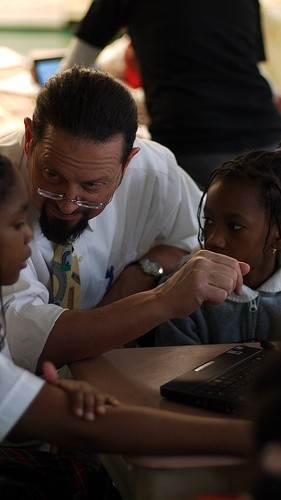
[52,340,281,500]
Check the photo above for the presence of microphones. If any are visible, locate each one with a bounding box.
[260,339,274,350]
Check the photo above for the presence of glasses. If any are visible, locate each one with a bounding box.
[32,114,122,209]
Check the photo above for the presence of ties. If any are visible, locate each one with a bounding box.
[52,239,81,309]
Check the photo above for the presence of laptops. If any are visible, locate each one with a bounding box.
[161,344,265,415]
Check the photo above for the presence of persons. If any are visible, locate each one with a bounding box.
[0,153,256,500]
[0,66,251,377]
[149,141,281,348]
[63,0,281,195]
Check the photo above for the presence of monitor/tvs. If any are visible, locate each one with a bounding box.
[33,56,64,86]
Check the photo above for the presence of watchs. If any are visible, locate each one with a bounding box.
[139,255,167,280]
